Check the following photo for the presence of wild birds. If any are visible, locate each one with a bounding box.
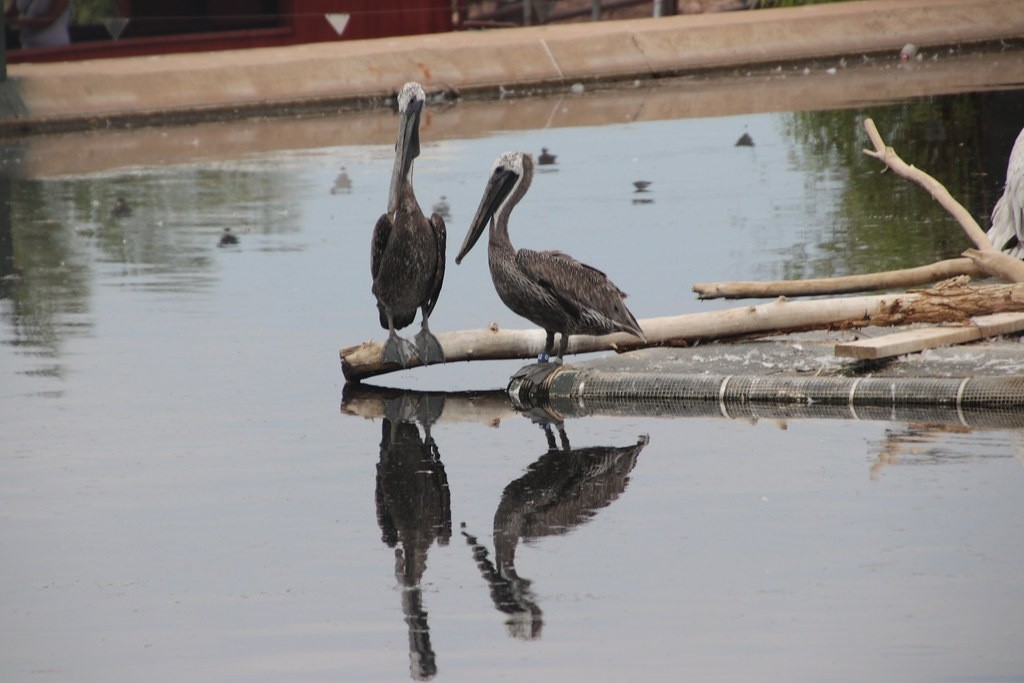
[370,80,449,369]
[109,124,756,247]
[375,392,452,683]
[461,409,650,640]
[454,149,648,387]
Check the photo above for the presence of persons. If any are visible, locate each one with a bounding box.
[2,0,74,49]
